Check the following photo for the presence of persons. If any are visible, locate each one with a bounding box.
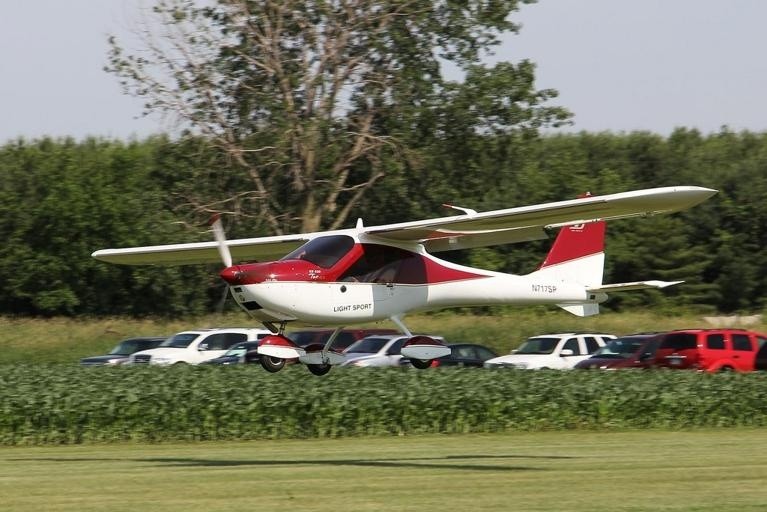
[341,243,407,284]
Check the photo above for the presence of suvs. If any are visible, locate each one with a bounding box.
[477,329,621,371]
[128,325,277,366]
[651,327,766,375]
[284,327,400,365]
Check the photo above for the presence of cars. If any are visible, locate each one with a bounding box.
[398,341,499,368]
[198,340,261,365]
[571,330,669,369]
[77,335,170,367]
[332,333,450,369]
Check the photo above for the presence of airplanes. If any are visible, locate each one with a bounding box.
[87,181,725,377]
